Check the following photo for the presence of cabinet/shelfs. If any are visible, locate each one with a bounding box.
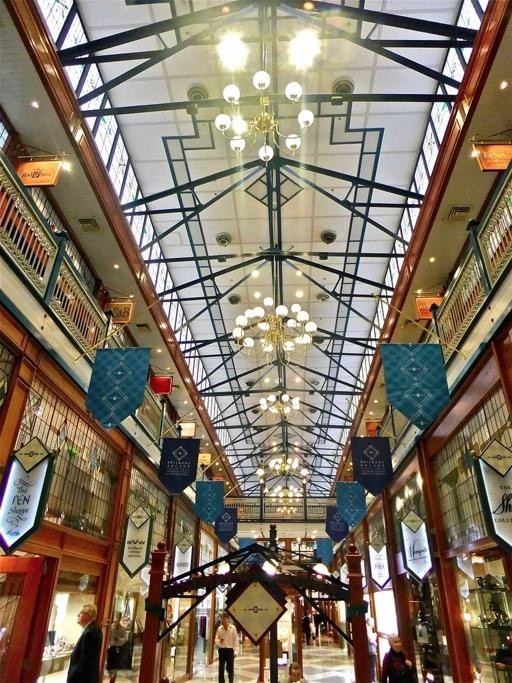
[464,585,512,682]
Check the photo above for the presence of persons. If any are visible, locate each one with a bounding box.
[303,610,324,647]
[366,617,413,682]
[215,615,239,683]
[106,611,127,683]
[66,604,103,682]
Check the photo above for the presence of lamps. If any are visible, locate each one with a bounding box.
[232,248,317,366]
[214,72,315,167]
[257,441,309,515]
[260,365,299,415]
